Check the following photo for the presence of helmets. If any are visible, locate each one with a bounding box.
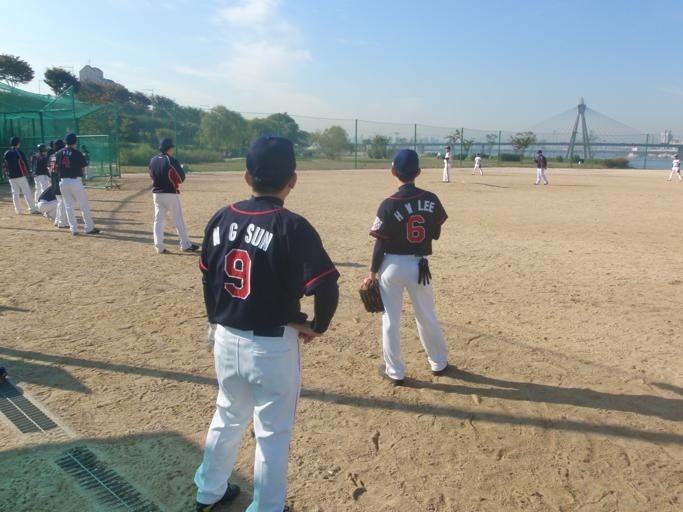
[37,144,46,151]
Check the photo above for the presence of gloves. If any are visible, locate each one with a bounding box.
[418,258,432,285]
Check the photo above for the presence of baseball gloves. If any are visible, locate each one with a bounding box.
[360,280,383,313]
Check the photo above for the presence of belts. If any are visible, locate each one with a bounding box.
[253,327,285,338]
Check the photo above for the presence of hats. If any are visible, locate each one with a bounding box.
[246,136,296,183]
[161,137,175,148]
[50,133,77,149]
[11,137,20,146]
[392,149,418,173]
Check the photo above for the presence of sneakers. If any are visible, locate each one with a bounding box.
[182,244,199,252]
[378,366,403,385]
[195,482,241,511]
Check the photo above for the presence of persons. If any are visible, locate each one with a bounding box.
[193,136,338,512]
[148,138,201,255]
[3,134,101,235]
[472,153,484,176]
[363,150,450,383]
[442,146,453,183]
[532,149,549,186]
[666,154,683,182]
